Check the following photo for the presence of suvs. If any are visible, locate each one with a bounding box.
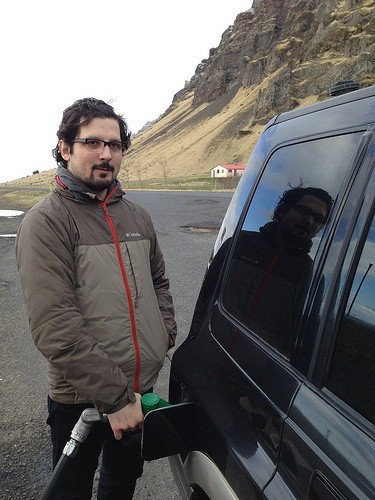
[141,82,374,500]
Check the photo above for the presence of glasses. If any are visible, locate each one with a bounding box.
[292,204,327,225]
[62,138,127,155]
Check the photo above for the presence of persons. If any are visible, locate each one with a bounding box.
[15,97,176,500]
[206,185,334,383]
